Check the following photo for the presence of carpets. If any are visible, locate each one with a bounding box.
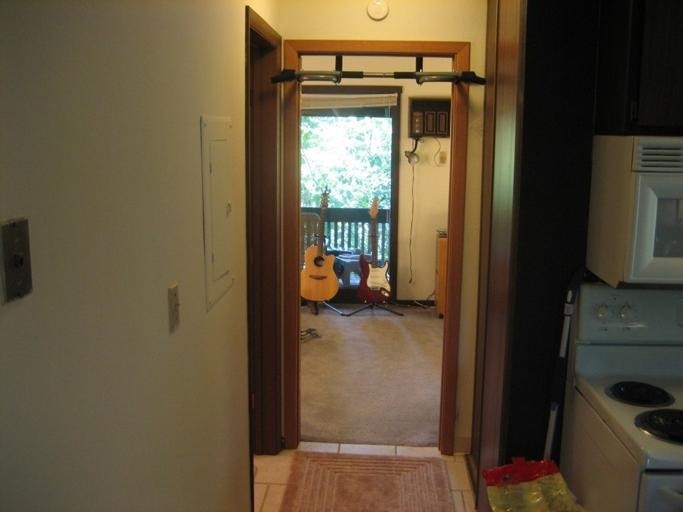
[278,451,456,512]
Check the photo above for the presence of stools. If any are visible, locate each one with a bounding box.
[335,253,360,287]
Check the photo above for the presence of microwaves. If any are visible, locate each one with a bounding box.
[585,135,683,290]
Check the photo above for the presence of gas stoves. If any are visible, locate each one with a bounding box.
[574,374,683,469]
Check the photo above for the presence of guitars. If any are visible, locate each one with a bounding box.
[299,185,340,302]
[357,196,394,304]
